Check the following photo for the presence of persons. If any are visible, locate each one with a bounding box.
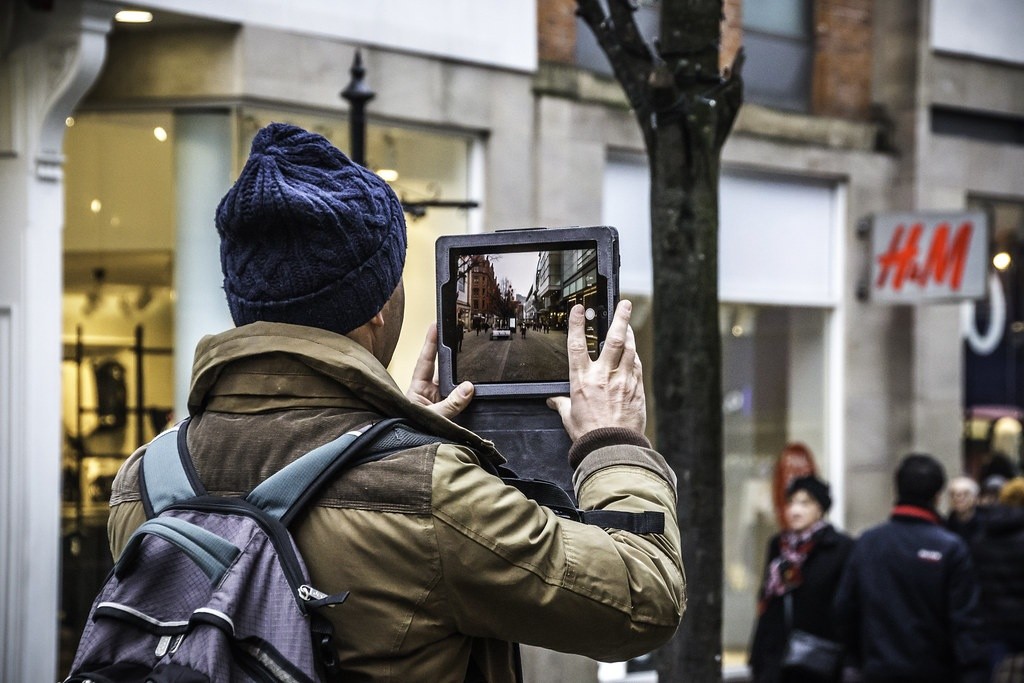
[745,442,855,683]
[971,476,1024,683]
[945,476,985,554]
[110,120,684,683]
[456,312,551,351]
[832,453,988,683]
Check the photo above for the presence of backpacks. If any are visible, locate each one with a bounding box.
[59,414,453,683]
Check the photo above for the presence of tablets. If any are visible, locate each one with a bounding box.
[435,226,621,396]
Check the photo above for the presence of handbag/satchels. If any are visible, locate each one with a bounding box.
[781,631,845,677]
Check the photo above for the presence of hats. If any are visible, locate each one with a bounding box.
[214,121,406,337]
